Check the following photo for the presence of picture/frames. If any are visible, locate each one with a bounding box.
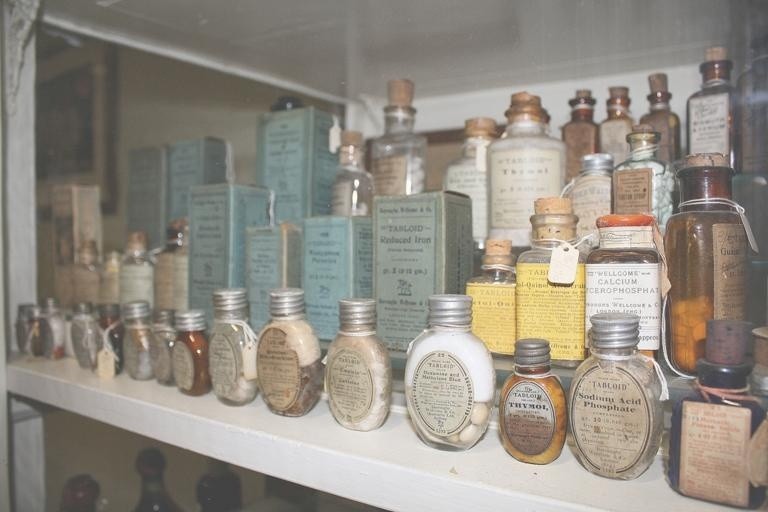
[34,37,120,216]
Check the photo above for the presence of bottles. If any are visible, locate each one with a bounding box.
[322,299,393,430]
[668,321,766,511]
[468,238,516,358]
[401,294,496,450]
[664,155,755,374]
[206,287,259,404]
[331,128,373,218]
[585,214,661,359]
[365,77,428,199]
[498,339,571,466]
[260,288,322,416]
[516,196,586,370]
[21,205,214,511]
[566,312,666,483]
[441,45,767,247]
